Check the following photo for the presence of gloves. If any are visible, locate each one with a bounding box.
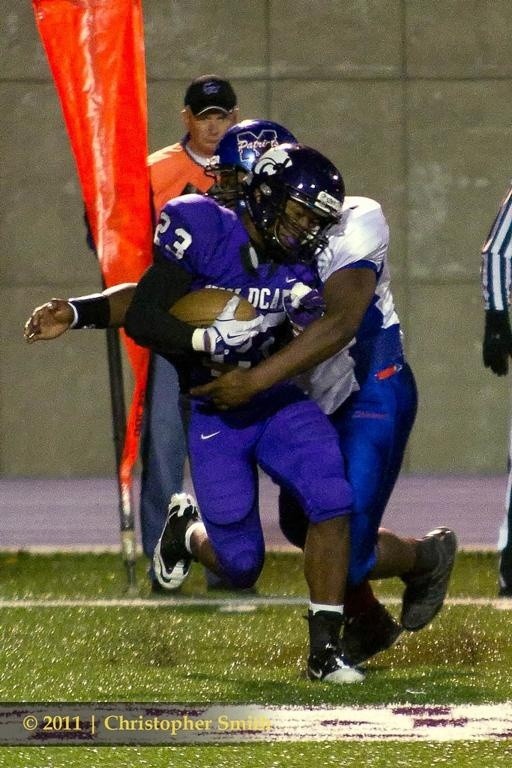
[205,295,265,355]
[284,281,327,325]
[484,310,512,376]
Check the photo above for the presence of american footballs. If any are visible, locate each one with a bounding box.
[168,288,256,329]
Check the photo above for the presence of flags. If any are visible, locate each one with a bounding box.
[34,0,154,489]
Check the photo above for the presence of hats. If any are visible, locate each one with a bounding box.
[185,74,237,117]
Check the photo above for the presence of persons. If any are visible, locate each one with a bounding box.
[23,117,455,665]
[478,181,512,609]
[140,75,258,599]
[124,142,367,687]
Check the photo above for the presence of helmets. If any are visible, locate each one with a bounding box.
[205,119,297,208]
[241,143,344,265]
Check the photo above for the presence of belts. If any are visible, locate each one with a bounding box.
[378,365,397,380]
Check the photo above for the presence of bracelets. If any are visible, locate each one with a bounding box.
[65,294,111,329]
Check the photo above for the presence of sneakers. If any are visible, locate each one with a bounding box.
[308,642,365,684]
[402,526,455,629]
[153,492,200,590]
[341,599,403,666]
